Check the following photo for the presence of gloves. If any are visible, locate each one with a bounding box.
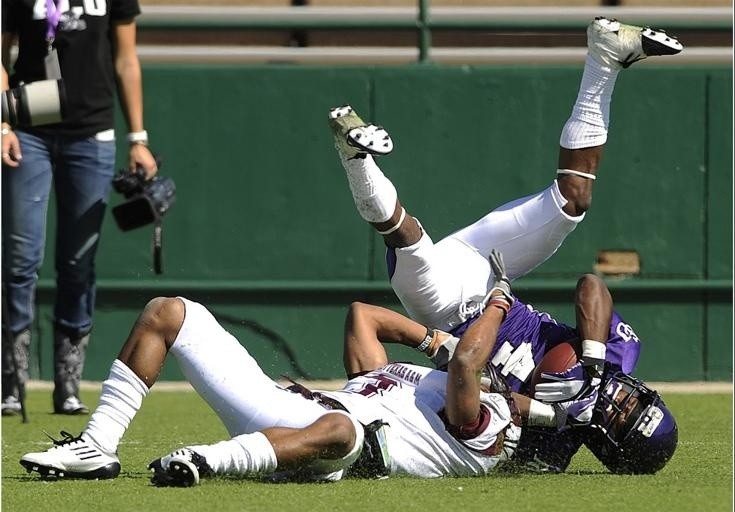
[485,246,516,307]
[554,389,600,435]
[423,326,461,371]
[534,355,607,402]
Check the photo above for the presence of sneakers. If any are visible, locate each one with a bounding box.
[18,428,122,481]
[328,101,395,160]
[585,16,685,72]
[144,444,212,488]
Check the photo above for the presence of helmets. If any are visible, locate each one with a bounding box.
[583,374,680,478]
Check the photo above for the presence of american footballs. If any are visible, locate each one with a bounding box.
[528,335,582,401]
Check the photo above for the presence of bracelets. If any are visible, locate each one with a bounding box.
[125,129,149,146]
[417,325,434,351]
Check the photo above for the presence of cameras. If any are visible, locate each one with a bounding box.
[1,77,67,132]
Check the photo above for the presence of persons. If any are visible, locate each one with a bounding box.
[2,0,160,416]
[328,16,683,475]
[19,248,525,487]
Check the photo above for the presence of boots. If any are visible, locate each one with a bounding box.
[44,328,91,416]
[1,329,33,417]
